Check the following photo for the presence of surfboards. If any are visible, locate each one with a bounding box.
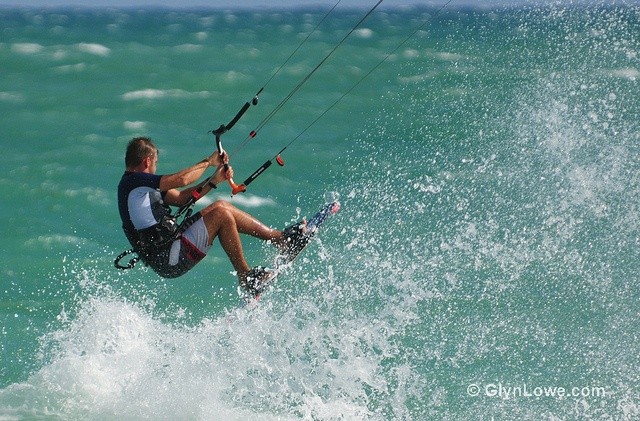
[242,201,339,306]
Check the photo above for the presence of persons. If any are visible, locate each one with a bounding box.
[117,138,307,298]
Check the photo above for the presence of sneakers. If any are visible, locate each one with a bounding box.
[275,217,306,254]
[240,266,273,292]
